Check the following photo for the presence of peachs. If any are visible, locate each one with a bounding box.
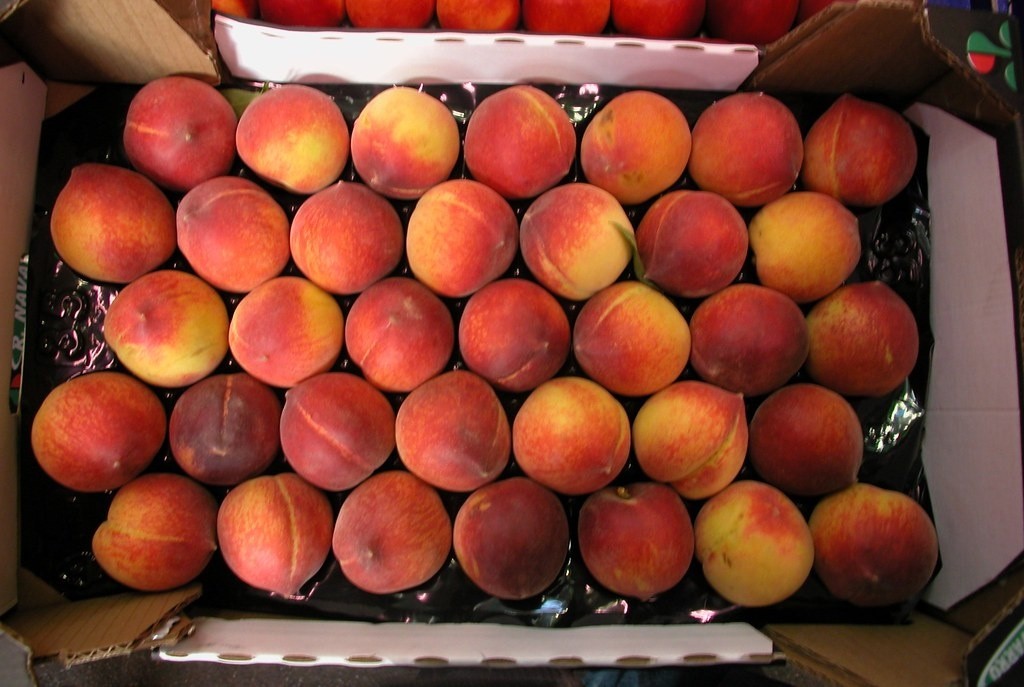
[209,0,835,48]
[31,77,939,610]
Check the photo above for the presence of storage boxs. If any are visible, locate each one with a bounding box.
[1,0,1024,687]
